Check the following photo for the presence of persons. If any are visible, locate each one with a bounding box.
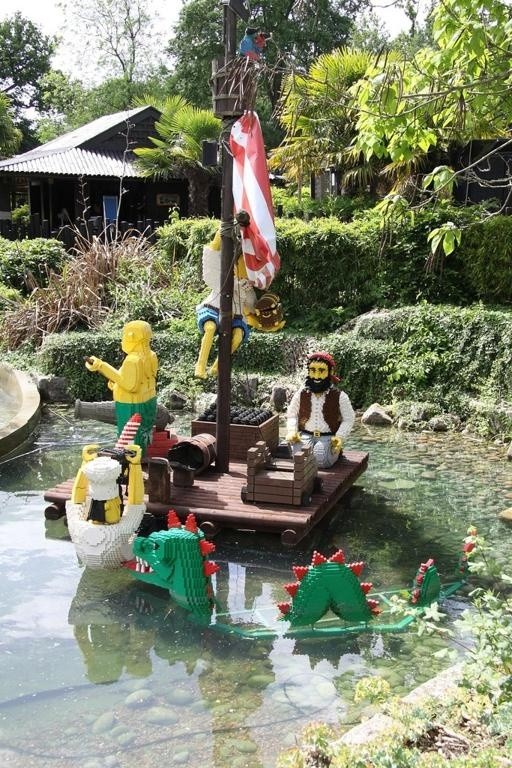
[237,23,265,62]
[82,318,159,458]
[64,444,148,569]
[284,358,359,467]
[191,228,251,378]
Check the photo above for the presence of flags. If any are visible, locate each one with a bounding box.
[226,110,280,289]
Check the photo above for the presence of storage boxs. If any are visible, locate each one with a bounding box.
[192,412,280,461]
